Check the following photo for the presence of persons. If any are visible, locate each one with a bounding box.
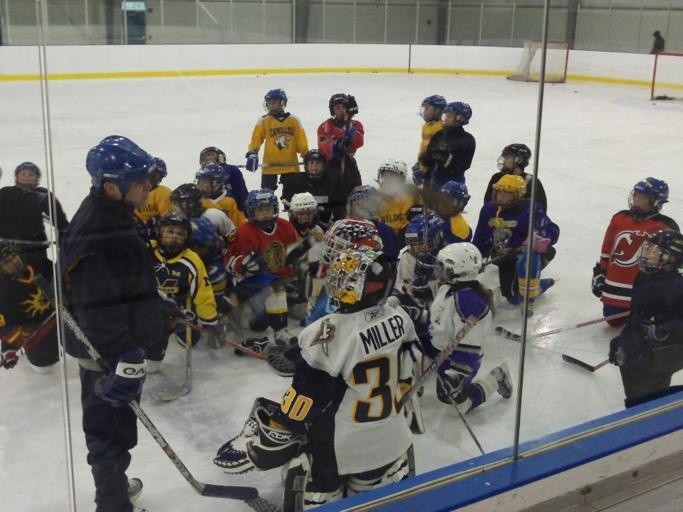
[417,101,476,203]
[246,248,424,511]
[649,31,664,53]
[316,92,364,208]
[472,173,559,302]
[53,134,170,511]
[245,88,309,210]
[0,238,63,369]
[0,161,70,260]
[483,143,546,214]
[421,241,512,416]
[591,176,679,328]
[607,228,682,405]
[411,94,445,185]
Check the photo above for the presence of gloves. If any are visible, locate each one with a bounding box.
[333,137,349,156]
[197,315,230,351]
[92,343,148,407]
[244,151,261,172]
[236,245,263,275]
[590,264,610,299]
[343,120,356,141]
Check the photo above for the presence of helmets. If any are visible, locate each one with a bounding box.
[435,240,483,284]
[302,149,326,179]
[419,94,447,122]
[346,185,381,219]
[291,192,318,228]
[13,160,42,185]
[200,147,226,166]
[495,142,532,170]
[376,159,407,190]
[0,239,28,281]
[84,135,158,196]
[494,173,527,209]
[187,215,216,246]
[158,214,193,258]
[329,93,351,117]
[245,186,280,228]
[405,209,446,256]
[444,101,472,125]
[636,227,683,276]
[319,247,400,307]
[264,88,288,113]
[169,183,202,216]
[437,180,470,217]
[150,158,168,187]
[320,217,384,265]
[194,163,232,200]
[626,176,670,215]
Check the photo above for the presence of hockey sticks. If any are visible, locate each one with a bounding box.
[562,353,611,372]
[158,291,193,402]
[247,314,480,512]
[494,310,632,342]
[59,307,258,500]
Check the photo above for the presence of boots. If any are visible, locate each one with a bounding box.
[127,475,144,498]
[489,366,512,401]
